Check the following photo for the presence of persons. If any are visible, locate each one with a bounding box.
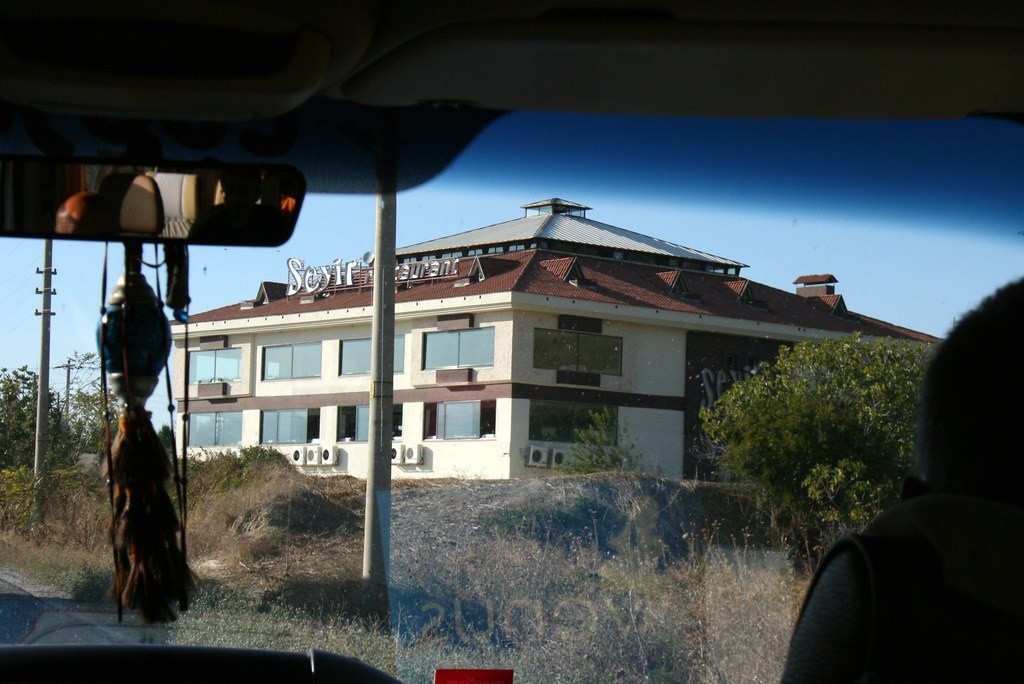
[186,170,281,243]
[54,192,117,235]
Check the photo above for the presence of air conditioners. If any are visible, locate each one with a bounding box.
[524,445,570,470]
[289,445,338,465]
[391,443,423,465]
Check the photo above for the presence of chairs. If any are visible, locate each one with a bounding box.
[781,282,1024,684]
